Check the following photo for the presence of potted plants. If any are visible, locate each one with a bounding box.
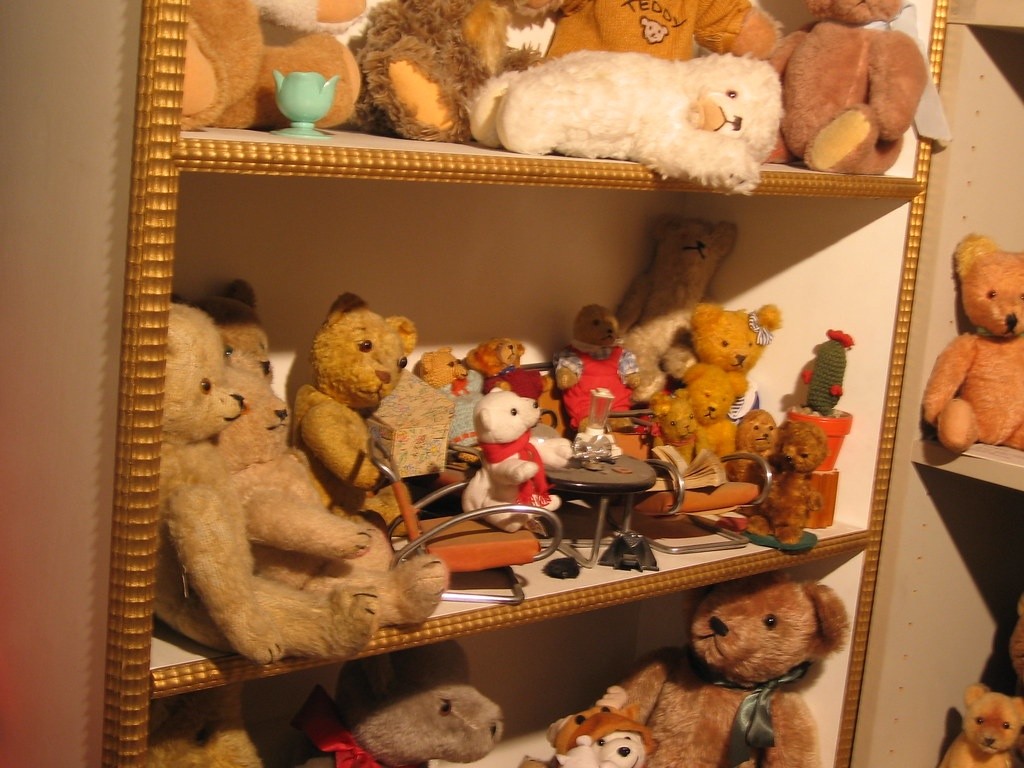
[788,328,854,469]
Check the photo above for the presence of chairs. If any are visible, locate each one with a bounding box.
[436,441,482,487]
[371,455,561,606]
[604,408,772,554]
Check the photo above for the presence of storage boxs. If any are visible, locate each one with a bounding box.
[364,369,456,478]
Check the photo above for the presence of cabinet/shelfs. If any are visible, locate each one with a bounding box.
[99,0,1024,768]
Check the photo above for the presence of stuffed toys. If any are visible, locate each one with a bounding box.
[138,577,847,767]
[183,0,950,196]
[922,233,1024,452]
[937,594,1024,768]
[150,216,848,663]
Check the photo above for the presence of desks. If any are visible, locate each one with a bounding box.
[540,455,658,567]
